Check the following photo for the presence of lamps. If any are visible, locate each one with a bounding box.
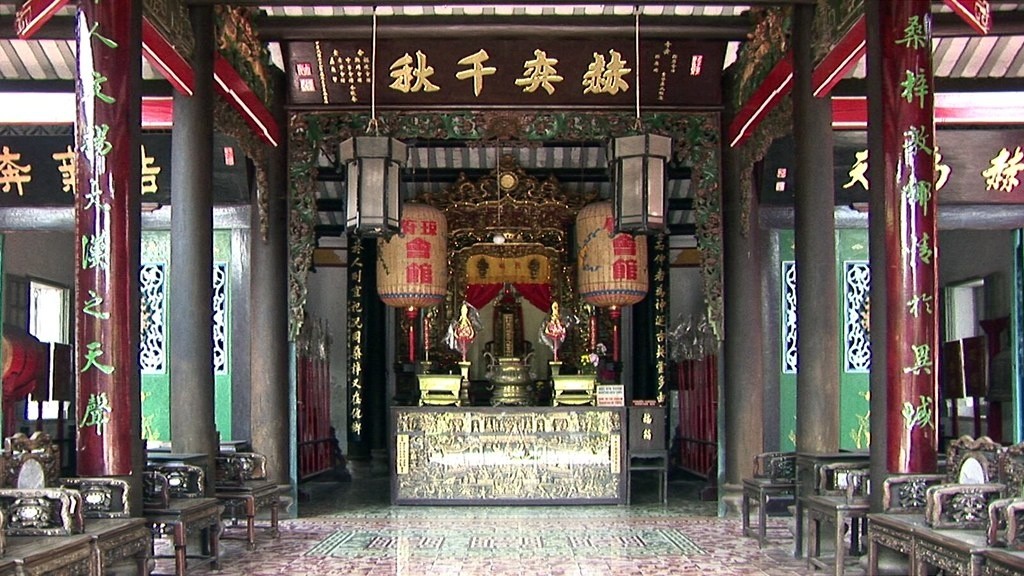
[608,5,675,238]
[336,4,409,241]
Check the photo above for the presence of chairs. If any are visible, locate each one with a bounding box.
[739,437,1024,576]
[0,417,292,576]
[626,405,668,507]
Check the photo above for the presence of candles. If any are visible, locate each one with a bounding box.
[424,317,429,351]
[591,314,597,350]
